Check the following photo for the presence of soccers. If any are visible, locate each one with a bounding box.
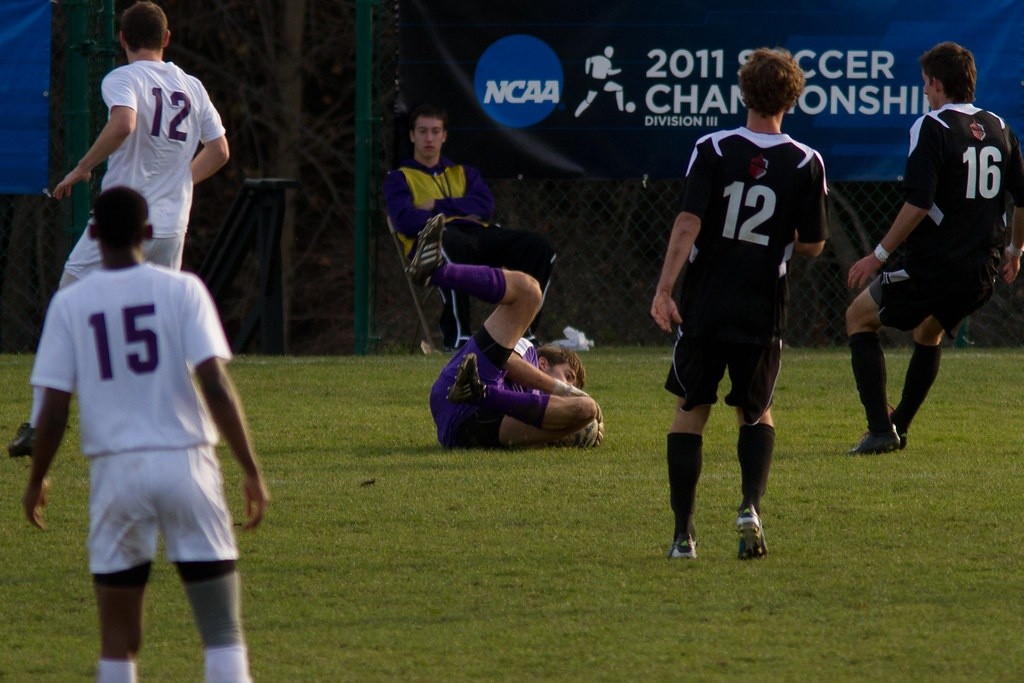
[544,417,598,450]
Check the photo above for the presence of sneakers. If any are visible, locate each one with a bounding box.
[445,352,482,404]
[405,213,448,290]
[845,427,900,455]
[888,413,907,449]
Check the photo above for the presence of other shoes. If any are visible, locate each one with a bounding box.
[8,422,35,455]
[736,503,768,561]
[669,534,698,558]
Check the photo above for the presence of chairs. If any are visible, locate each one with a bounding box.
[386,217,440,358]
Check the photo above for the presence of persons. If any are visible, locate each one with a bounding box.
[652,50,831,561]
[381,101,557,349]
[847,41,1024,458]
[24,186,269,683]
[7,3,228,458]
[404,212,605,450]
